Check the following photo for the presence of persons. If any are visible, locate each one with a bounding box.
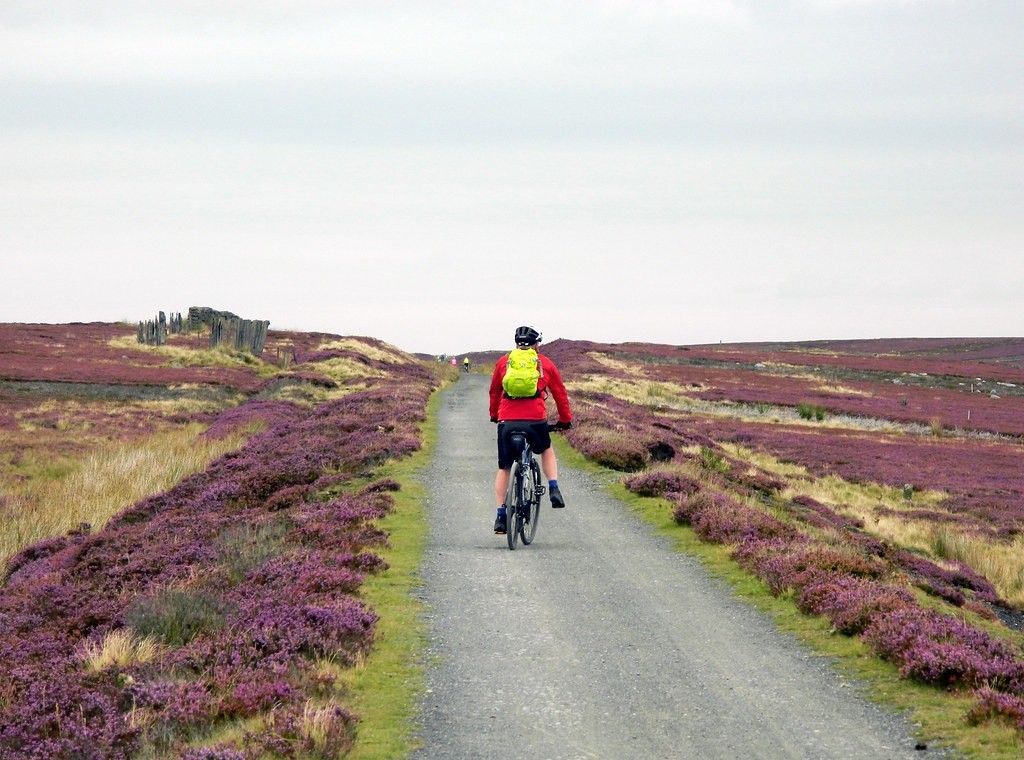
[450,356,458,367]
[489,325,573,532]
[463,356,470,373]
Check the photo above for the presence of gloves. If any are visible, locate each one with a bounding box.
[554,421,572,432]
[491,418,499,424]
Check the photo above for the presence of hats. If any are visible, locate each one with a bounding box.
[515,326,542,346]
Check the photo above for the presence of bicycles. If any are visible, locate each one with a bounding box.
[463,364,469,373]
[490,418,573,550]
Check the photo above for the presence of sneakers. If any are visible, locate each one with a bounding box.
[494,514,507,531]
[549,485,565,508]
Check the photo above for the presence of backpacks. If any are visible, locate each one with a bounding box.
[500,348,540,398]
